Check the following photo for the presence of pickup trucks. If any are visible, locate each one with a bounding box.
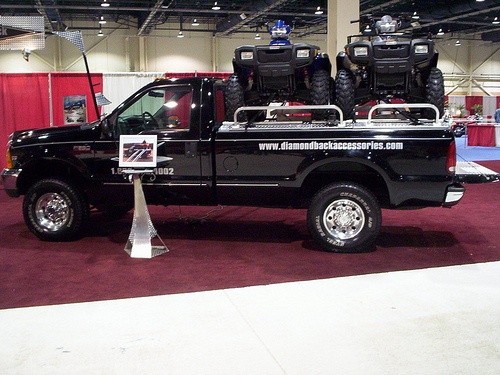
[0,74,500,254]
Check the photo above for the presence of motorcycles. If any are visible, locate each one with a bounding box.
[222,18,336,121]
[331,12,446,119]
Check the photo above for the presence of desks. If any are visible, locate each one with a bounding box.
[467,122,500,147]
